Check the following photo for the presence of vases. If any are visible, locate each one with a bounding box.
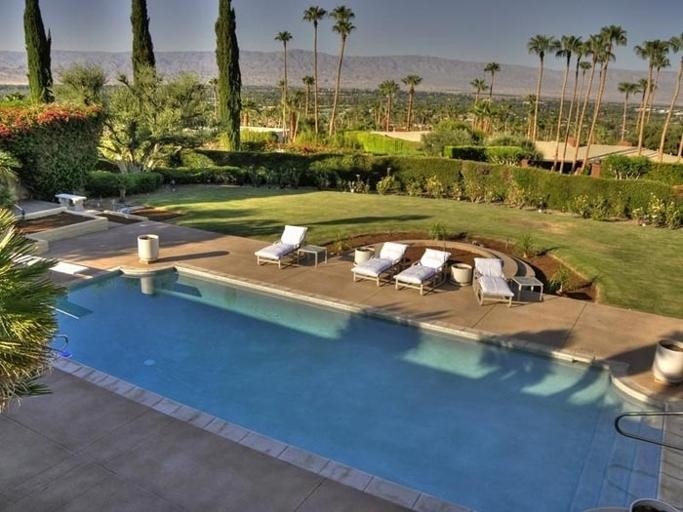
[137,233,159,265]
[448,263,473,287]
[352,246,375,267]
[650,338,683,389]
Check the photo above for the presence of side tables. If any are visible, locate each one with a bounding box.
[511,276,544,303]
[297,245,328,268]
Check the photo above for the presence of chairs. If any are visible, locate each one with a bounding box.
[474,257,515,307]
[352,240,409,288]
[393,248,451,296]
[255,224,309,269]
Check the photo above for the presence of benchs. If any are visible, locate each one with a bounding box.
[54,193,87,211]
